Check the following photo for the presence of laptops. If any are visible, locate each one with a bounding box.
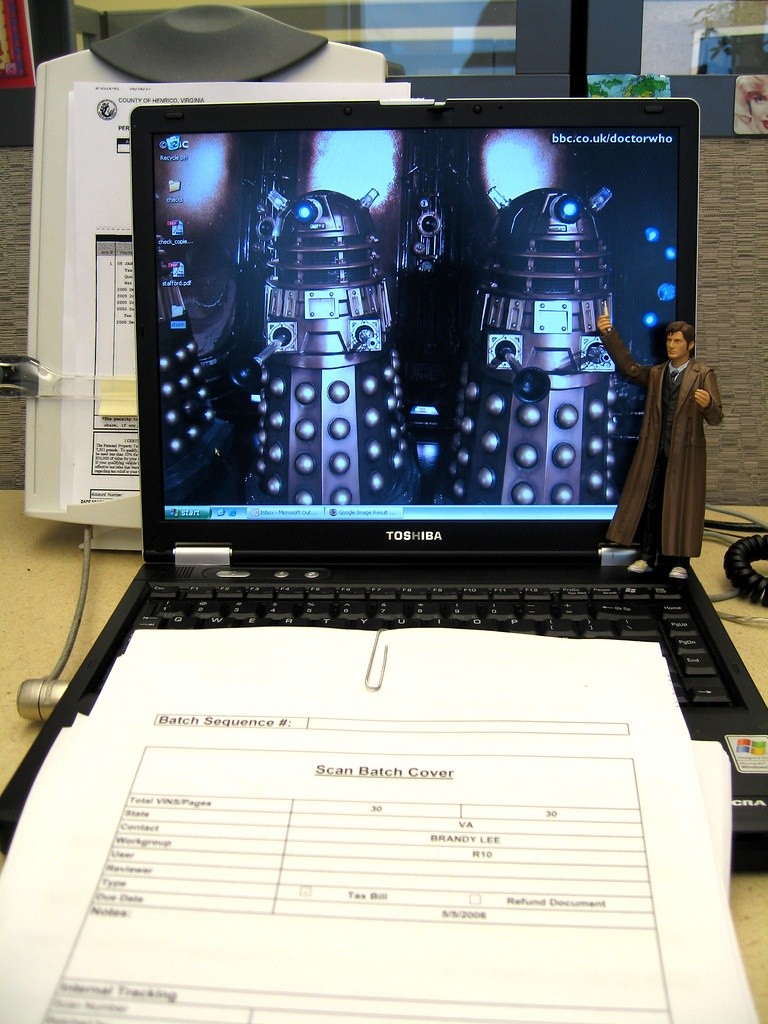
[0,99,768,877]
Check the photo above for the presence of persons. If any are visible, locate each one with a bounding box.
[595,315,724,579]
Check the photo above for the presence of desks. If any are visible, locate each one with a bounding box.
[1,489,768,1023]
